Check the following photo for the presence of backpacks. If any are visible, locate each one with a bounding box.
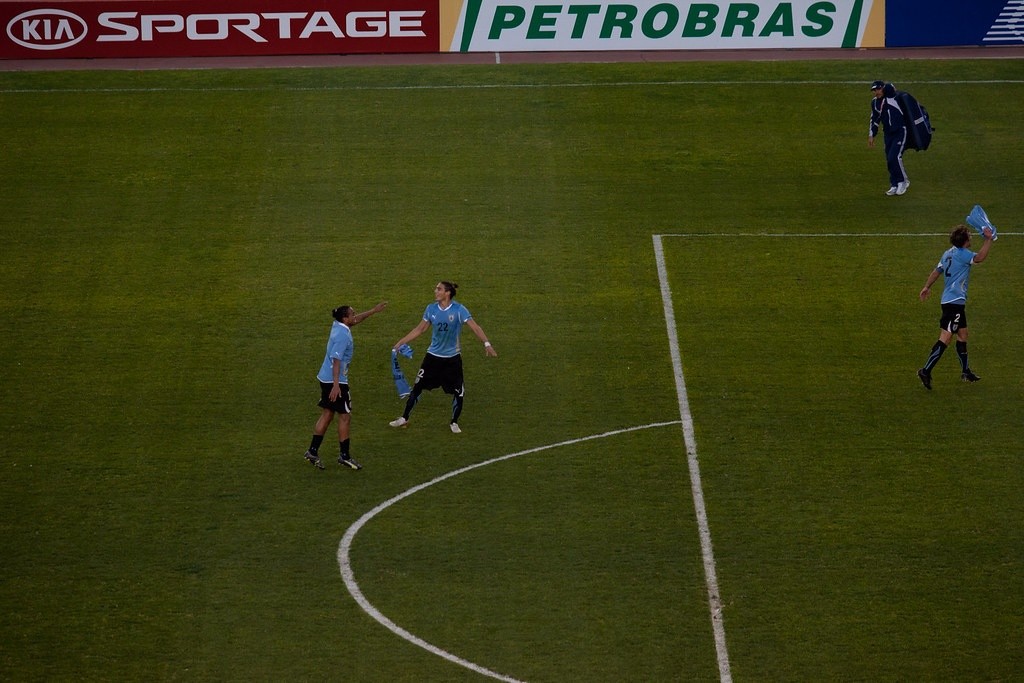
[894,89,932,153]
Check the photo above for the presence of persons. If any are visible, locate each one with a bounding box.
[868,80,935,196]
[389,281,497,434]
[917,205,998,390]
[303,301,387,470]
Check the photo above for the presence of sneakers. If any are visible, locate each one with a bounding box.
[390,415,411,429]
[450,422,460,433]
[337,455,362,470]
[919,369,933,392]
[886,187,898,196]
[961,371,980,382]
[896,180,911,195]
[304,451,325,470]
